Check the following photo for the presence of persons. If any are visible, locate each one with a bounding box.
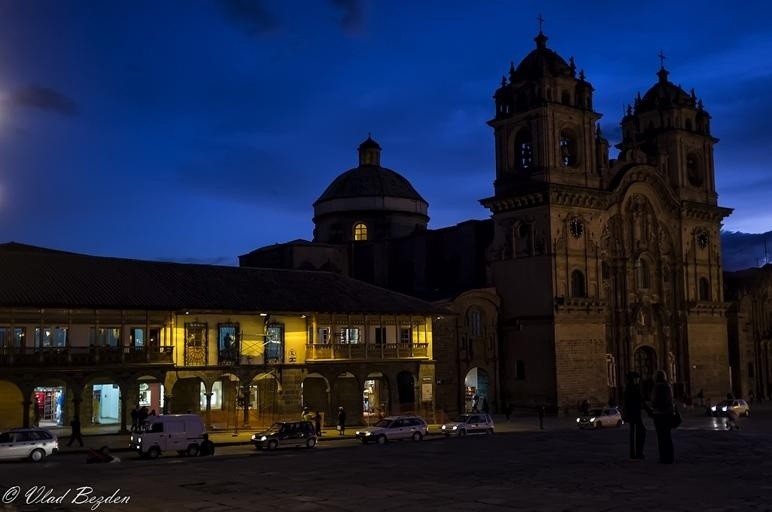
[700,388,704,407]
[223,332,233,348]
[337,406,346,436]
[130,404,157,434]
[724,403,745,430]
[362,397,371,413]
[620,368,676,464]
[468,387,480,414]
[34,397,41,427]
[66,415,84,448]
[301,407,322,438]
[538,404,545,430]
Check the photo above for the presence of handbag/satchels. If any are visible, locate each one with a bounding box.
[663,412,682,428]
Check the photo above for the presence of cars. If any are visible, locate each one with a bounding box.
[251,420,318,449]
[576,407,622,428]
[356,416,427,445]
[441,413,494,437]
[711,399,750,417]
[0,428,59,462]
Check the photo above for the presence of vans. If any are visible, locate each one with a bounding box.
[129,414,205,460]
